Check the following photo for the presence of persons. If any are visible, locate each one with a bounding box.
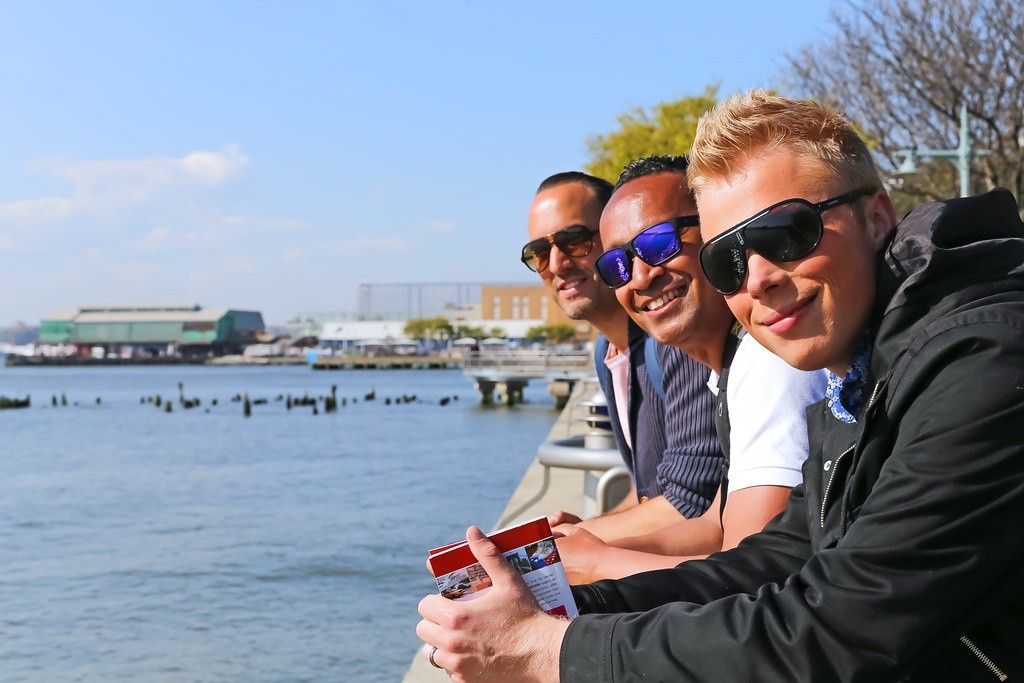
[416,92,1024,683]
[520,153,830,587]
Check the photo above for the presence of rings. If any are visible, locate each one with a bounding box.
[429,646,444,670]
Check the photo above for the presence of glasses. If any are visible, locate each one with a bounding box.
[521,224,599,273]
[594,214,700,289]
[697,187,874,295]
